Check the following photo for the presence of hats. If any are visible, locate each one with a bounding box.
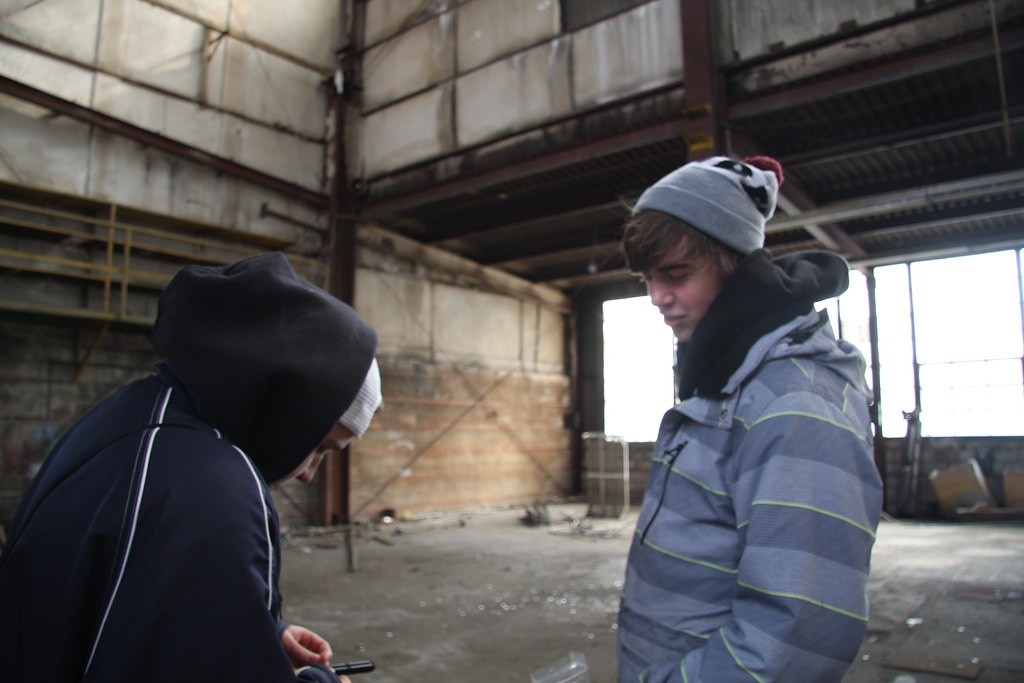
[631,150,785,256]
[338,357,383,441]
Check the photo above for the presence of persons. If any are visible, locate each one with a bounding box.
[0,249,380,682]
[615,156,885,678]
